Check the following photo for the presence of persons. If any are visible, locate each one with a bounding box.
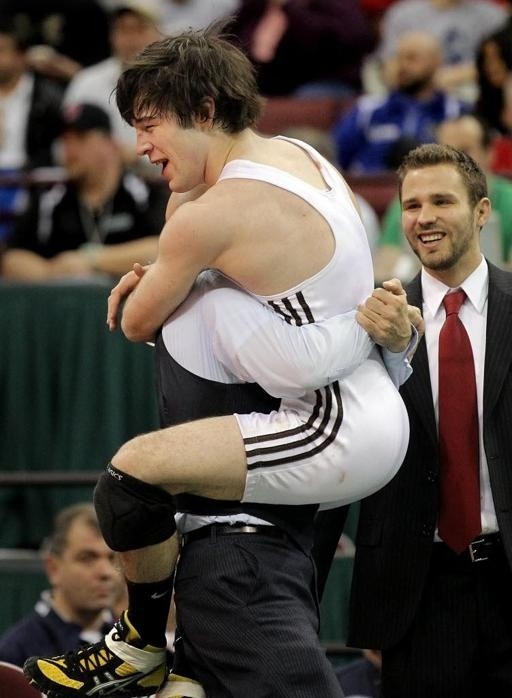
[22,14,412,698]
[1,500,126,669]
[154,184,425,698]
[347,142,512,697]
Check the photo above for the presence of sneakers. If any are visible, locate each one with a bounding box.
[20,611,169,697]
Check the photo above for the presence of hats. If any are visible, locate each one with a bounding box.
[54,102,111,138]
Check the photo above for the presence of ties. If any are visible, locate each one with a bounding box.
[439,288,482,551]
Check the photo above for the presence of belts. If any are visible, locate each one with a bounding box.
[430,530,503,566]
